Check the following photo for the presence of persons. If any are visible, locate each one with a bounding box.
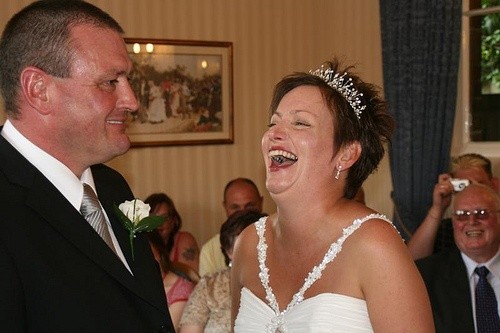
[406,153,500,333]
[0,0,176,333]
[143,177,269,333]
[231,54,436,333]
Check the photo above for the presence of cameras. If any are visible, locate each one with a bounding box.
[451,179,470,192]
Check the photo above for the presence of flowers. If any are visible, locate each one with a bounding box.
[113,198,167,262]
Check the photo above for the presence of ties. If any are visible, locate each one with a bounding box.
[474,266,500,333]
[80,182,117,255]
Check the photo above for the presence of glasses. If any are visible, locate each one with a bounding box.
[452,208,500,221]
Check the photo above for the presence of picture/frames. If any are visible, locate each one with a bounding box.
[115,38,233,148]
